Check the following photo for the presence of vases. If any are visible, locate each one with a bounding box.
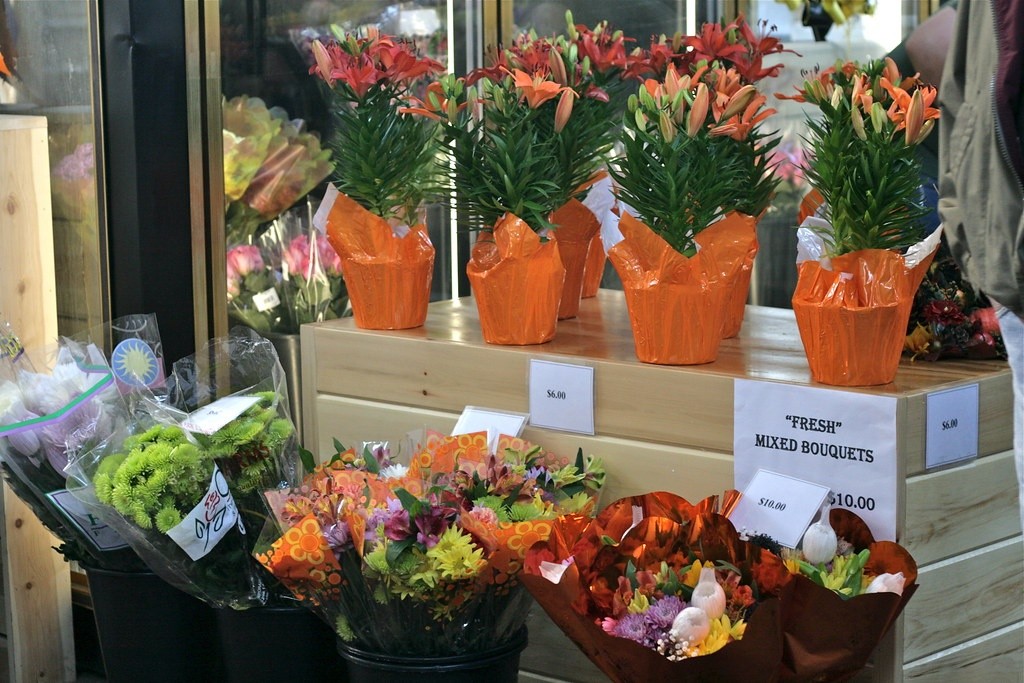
[625,284,721,366]
[552,238,584,322]
[337,253,433,330]
[580,227,610,297]
[470,263,566,346]
[792,295,917,384]
[693,257,752,339]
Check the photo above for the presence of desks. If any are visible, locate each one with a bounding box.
[297,288,1023,682]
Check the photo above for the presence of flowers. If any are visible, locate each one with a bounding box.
[773,53,944,267]
[399,24,639,246]
[307,21,481,227]
[0,336,924,683]
[602,62,785,264]
[51,96,348,334]
[482,6,805,236]
